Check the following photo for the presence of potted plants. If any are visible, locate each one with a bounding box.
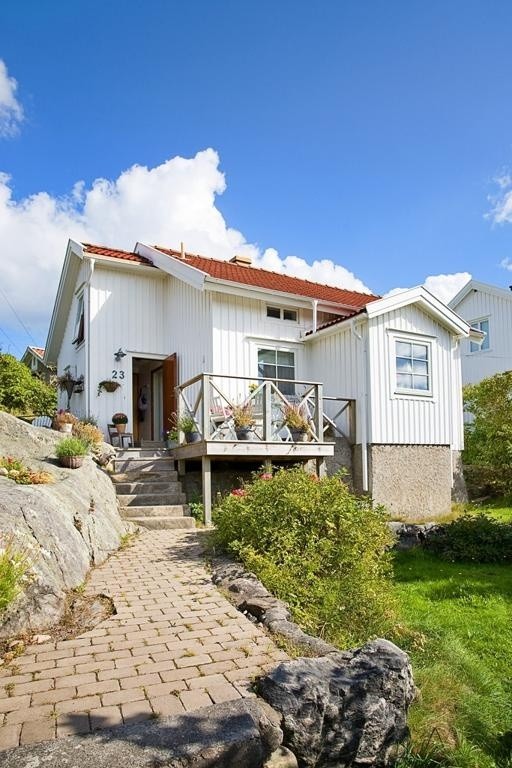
[97,381,120,397]
[171,409,200,443]
[55,413,102,468]
[166,429,179,449]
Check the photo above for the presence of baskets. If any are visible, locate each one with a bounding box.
[59,454,84,469]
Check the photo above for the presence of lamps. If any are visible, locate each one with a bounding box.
[115,348,128,361]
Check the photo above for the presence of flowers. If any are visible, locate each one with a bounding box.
[112,413,128,424]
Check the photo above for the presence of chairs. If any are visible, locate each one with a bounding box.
[210,393,310,441]
[107,424,134,448]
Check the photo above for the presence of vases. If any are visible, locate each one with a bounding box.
[116,425,126,433]
